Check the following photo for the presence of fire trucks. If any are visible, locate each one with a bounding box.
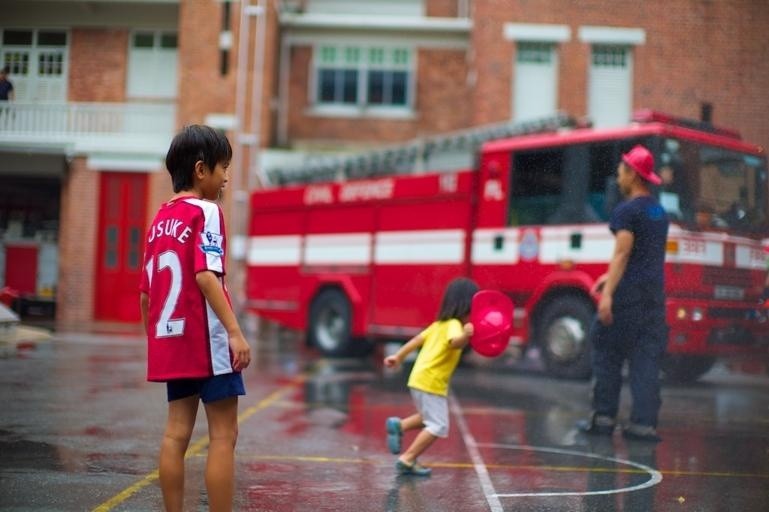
[243,107,769,382]
[0,218,60,320]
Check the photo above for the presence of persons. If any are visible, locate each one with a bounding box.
[140,124,251,512]
[577,144,669,440]
[383,279,481,475]
[0,70,15,100]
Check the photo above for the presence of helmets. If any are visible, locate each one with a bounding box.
[622,144,664,186]
[468,290,515,357]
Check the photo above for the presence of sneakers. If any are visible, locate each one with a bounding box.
[386,414,402,454]
[576,413,615,435]
[395,460,432,477]
[621,425,656,442]
